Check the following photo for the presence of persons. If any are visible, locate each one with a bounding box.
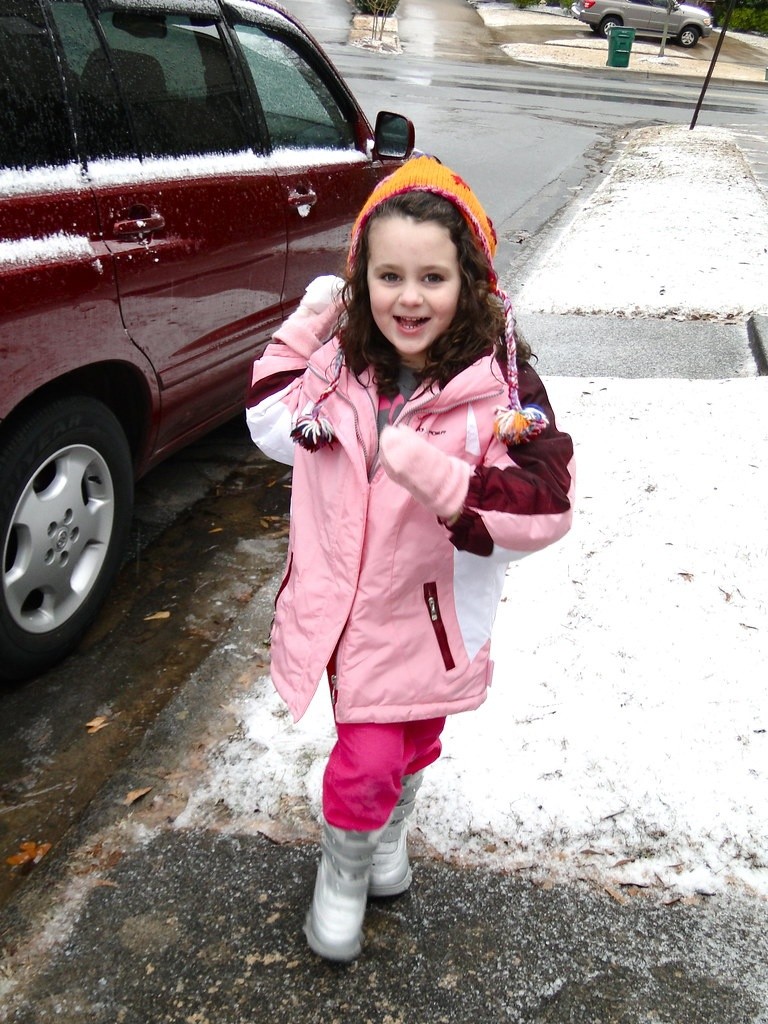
[246,157,574,962]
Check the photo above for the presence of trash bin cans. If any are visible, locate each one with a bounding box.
[606,26,636,67]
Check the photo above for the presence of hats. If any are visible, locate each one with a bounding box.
[291,153,549,453]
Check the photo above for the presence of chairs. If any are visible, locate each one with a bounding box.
[79,51,167,155]
[0,31,71,146]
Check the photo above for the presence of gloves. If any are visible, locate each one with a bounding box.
[272,275,352,359]
[380,422,470,517]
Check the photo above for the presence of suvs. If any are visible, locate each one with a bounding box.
[0,0,413,684]
[568,0,712,47]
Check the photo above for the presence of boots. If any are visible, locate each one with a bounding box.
[305,809,392,960]
[368,769,425,894]
